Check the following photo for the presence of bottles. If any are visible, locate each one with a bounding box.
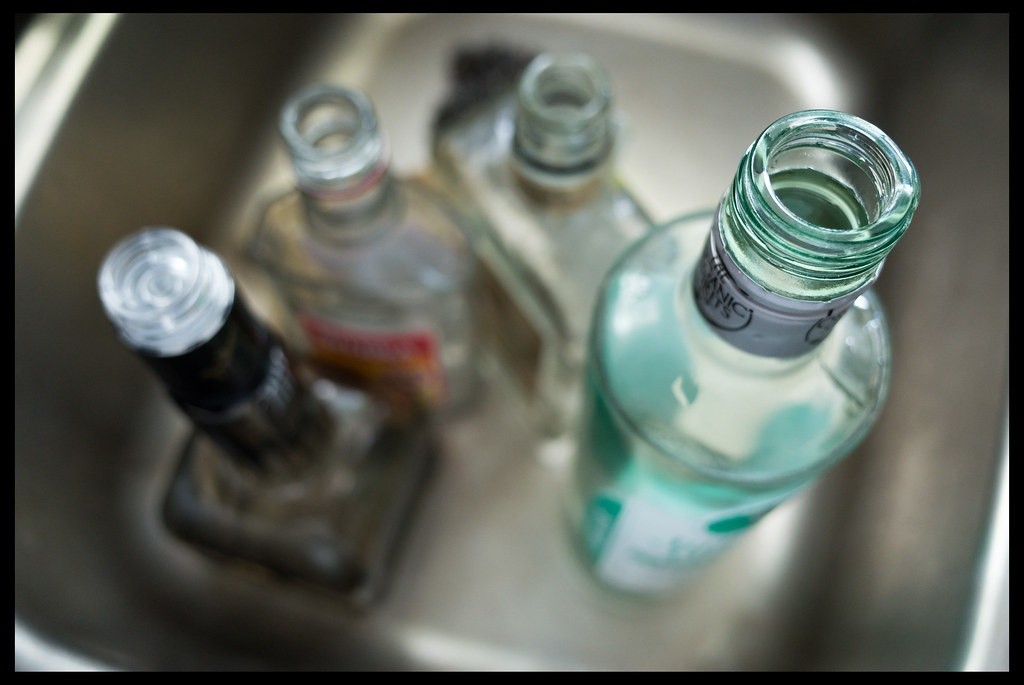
[94,225,444,617]
[237,84,490,418]
[558,107,925,604]
[426,41,684,438]
